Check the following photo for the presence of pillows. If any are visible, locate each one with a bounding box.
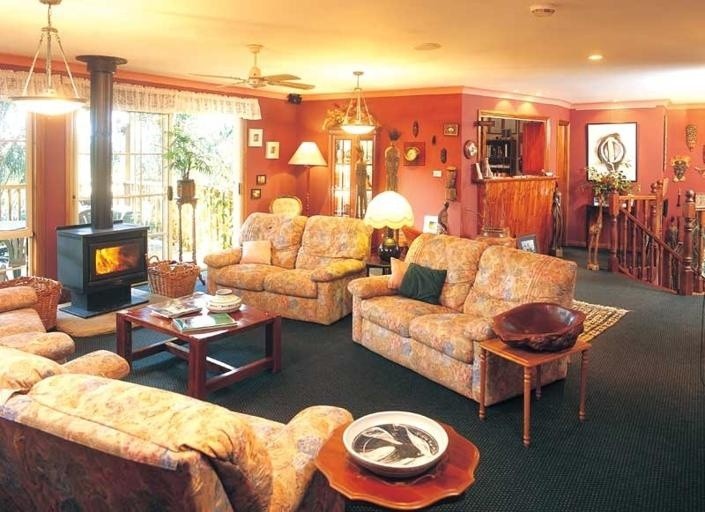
[387,255,408,290]
[238,240,271,264]
[399,264,446,305]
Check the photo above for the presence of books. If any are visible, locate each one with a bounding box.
[144,289,213,320]
[173,313,239,336]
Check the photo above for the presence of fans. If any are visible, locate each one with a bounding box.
[182,44,317,94]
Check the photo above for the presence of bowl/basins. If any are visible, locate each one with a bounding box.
[335,411,449,480]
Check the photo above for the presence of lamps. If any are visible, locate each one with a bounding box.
[340,70,377,136]
[287,140,328,217]
[9,0,89,117]
[362,191,416,260]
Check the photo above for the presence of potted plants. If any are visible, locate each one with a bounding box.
[151,113,221,198]
[586,166,639,204]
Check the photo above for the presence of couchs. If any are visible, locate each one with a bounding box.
[0,286,76,365]
[0,350,355,512]
[204,212,372,326]
[347,234,579,407]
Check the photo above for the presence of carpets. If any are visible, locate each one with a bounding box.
[572,299,629,346]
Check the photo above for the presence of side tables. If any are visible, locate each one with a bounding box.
[478,339,591,448]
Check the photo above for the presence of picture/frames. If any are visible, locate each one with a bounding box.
[587,123,637,182]
[251,188,260,198]
[264,141,279,159]
[257,174,266,184]
[443,123,458,135]
[247,128,263,148]
